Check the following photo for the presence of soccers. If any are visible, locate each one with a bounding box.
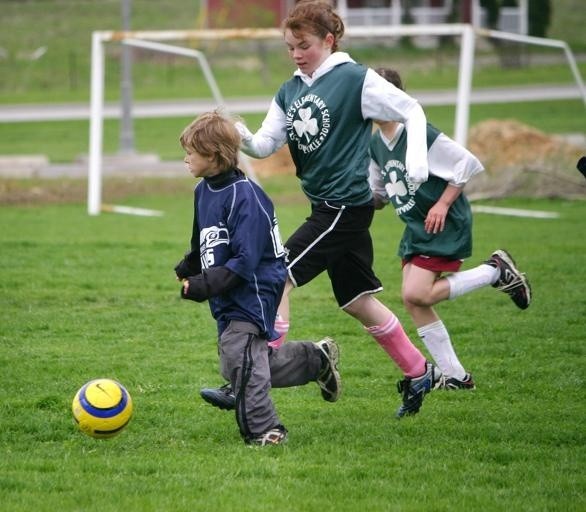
[72,378,134,438]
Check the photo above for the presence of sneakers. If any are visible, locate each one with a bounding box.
[394,360,443,420]
[245,427,289,449]
[432,373,477,392]
[200,383,236,411]
[314,336,343,403]
[483,249,532,311]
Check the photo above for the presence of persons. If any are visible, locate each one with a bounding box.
[367,68,530,392]
[199,1,444,419]
[171,107,341,448]
